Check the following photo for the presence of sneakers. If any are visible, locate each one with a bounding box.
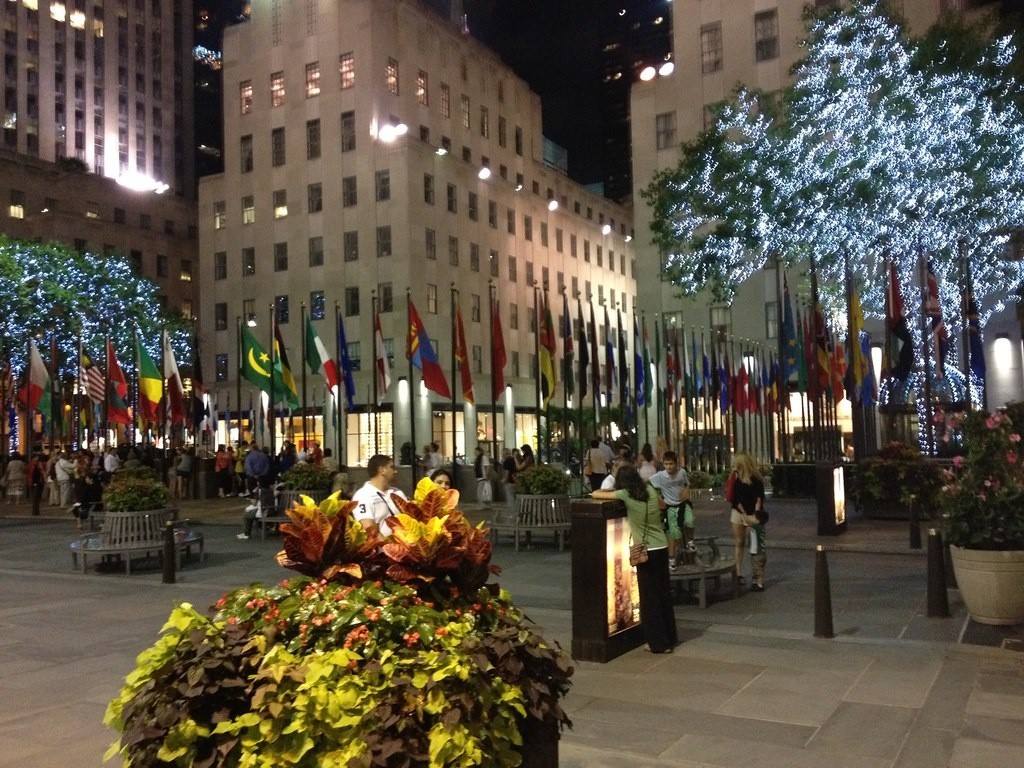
[236,533,250,539]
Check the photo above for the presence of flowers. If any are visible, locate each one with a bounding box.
[932,372,1024,549]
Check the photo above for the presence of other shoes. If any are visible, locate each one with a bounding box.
[750,583,765,591]
[643,645,671,653]
[687,539,697,552]
[737,576,746,584]
[668,558,676,571]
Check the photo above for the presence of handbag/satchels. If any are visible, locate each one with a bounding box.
[481,481,492,503]
[629,543,649,567]
[585,450,592,476]
[69,473,74,481]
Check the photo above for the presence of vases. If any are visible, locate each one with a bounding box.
[949,543,1024,626]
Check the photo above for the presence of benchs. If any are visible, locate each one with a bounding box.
[668,551,741,610]
[258,515,294,540]
[486,520,572,552]
[70,527,206,577]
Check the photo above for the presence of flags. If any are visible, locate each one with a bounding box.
[491,301,507,402]
[453,299,475,405]
[925,259,949,379]
[540,263,915,422]
[405,301,451,399]
[338,316,356,411]
[375,308,392,407]
[964,268,986,378]
[0,300,344,439]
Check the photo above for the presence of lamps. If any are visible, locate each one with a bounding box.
[433,411,442,416]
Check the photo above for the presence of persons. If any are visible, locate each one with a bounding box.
[431,469,453,491]
[352,455,407,540]
[73,472,105,529]
[730,454,769,592]
[644,451,697,570]
[332,465,349,500]
[601,455,631,490]
[0,441,336,509]
[502,444,534,509]
[636,443,660,479]
[474,448,491,511]
[237,475,269,539]
[593,466,679,653]
[586,436,615,492]
[423,442,441,470]
